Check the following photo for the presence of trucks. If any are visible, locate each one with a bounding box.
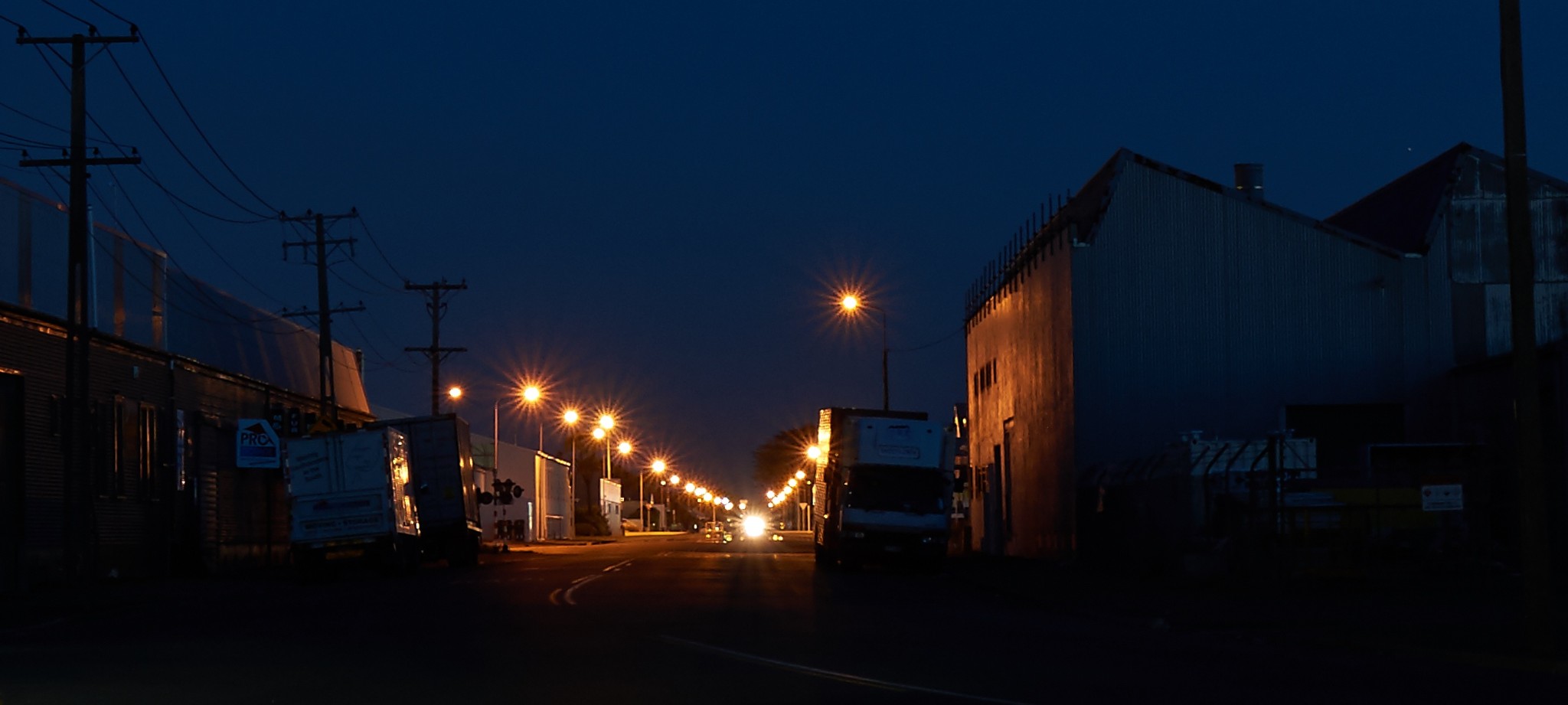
[280,411,485,573]
[812,406,960,585]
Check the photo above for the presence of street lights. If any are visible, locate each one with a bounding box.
[637,457,667,532]
[564,409,580,540]
[837,293,892,410]
[593,428,608,521]
[493,384,542,472]
[601,413,615,482]
